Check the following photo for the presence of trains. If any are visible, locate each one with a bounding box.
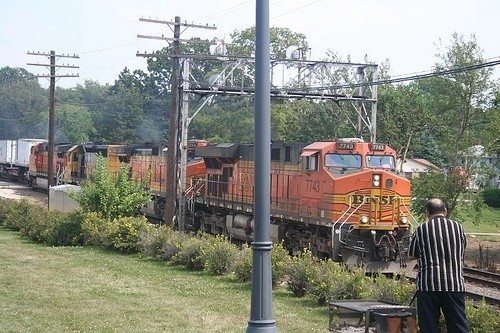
[0,136,419,281]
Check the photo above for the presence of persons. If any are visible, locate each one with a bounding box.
[407,198,470,333]
